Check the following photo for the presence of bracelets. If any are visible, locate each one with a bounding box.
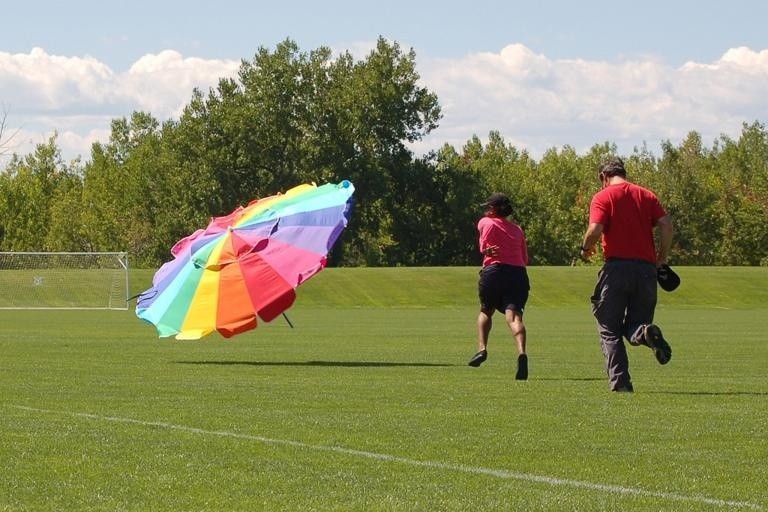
[580,246,592,252]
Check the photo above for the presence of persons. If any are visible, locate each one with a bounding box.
[468,196,531,382]
[576,157,672,395]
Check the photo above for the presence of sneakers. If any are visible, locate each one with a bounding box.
[516,354,528,380]
[644,324,671,364]
[617,383,633,392]
[469,350,487,367]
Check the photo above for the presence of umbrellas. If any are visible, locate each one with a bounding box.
[133,180,355,347]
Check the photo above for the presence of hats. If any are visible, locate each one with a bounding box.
[657,264,680,291]
[480,194,510,209]
